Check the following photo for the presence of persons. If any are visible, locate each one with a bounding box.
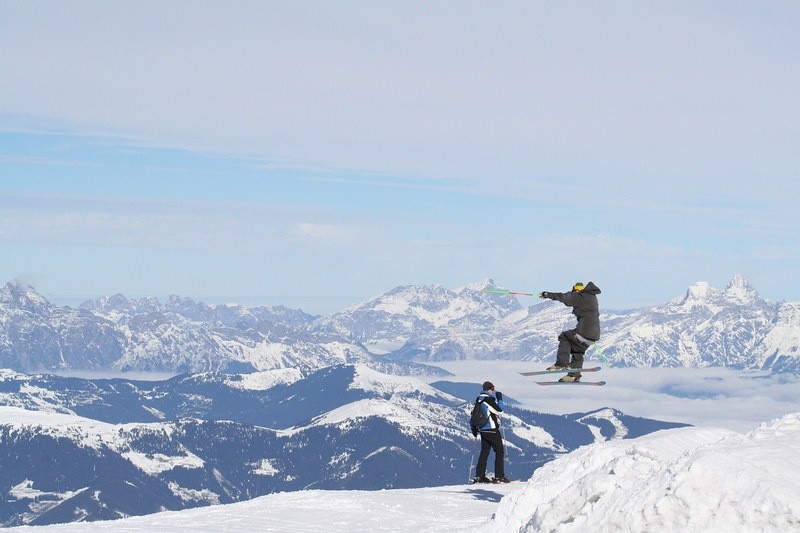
[538,281,602,384]
[472,381,510,484]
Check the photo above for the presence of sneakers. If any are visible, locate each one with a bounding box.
[546,364,568,370]
[558,374,582,382]
[473,475,493,484]
[492,477,511,484]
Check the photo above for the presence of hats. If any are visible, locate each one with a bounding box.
[482,381,495,391]
[573,282,585,291]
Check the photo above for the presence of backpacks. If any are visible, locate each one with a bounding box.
[470,396,498,429]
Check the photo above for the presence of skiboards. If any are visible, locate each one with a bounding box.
[517,365,608,387]
[464,479,529,487]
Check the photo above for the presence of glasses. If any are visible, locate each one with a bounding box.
[490,388,494,391]
